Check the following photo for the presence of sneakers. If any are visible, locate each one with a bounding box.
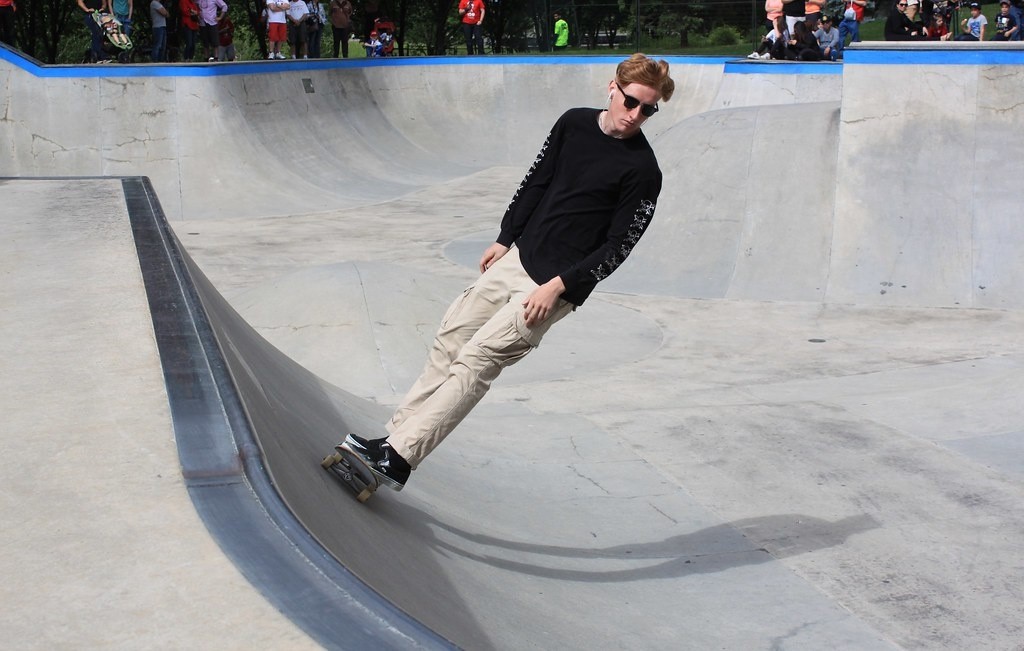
[341,439,411,491]
[268,52,274,60]
[340,433,390,451]
[747,51,760,59]
[760,53,771,60]
[275,51,286,60]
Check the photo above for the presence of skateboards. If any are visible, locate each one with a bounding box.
[320,441,384,504]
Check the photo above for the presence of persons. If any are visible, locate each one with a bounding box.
[343,54,676,487]
[0,0,1024,62]
[552,12,569,55]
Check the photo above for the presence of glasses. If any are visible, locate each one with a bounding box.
[900,3,908,7]
[615,81,659,117]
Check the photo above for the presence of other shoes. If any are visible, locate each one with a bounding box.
[96,59,112,64]
[125,43,131,46]
[117,41,121,45]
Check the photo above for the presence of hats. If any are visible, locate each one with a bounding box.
[1000,0,1011,8]
[370,30,378,36]
[968,1,981,10]
[820,14,831,23]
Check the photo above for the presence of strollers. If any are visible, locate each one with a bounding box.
[91,11,133,63]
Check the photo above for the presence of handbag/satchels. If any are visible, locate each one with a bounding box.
[843,0,856,21]
[306,19,320,34]
